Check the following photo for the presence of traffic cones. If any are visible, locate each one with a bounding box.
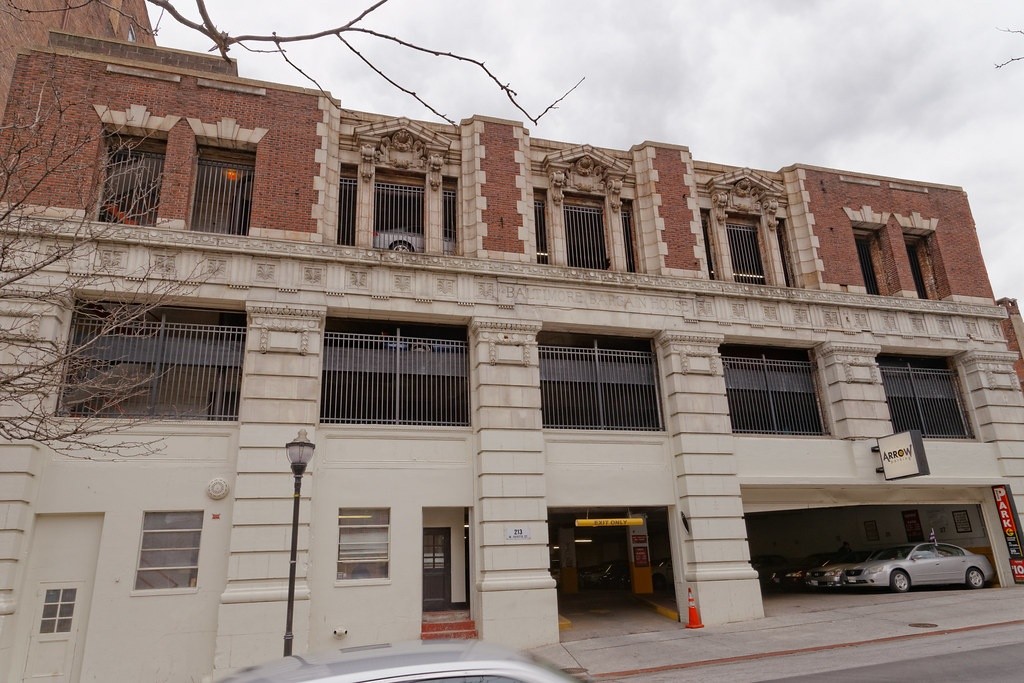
[684,587,705,628]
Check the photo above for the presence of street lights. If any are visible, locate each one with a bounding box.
[283,427,317,656]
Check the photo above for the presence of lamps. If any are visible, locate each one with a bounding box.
[224,166,244,181]
[207,477,229,500]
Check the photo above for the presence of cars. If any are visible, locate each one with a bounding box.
[807,548,873,592]
[752,563,780,595]
[784,552,833,593]
[844,542,997,594]
[371,225,456,253]
[577,559,674,588]
[208,639,593,683]
